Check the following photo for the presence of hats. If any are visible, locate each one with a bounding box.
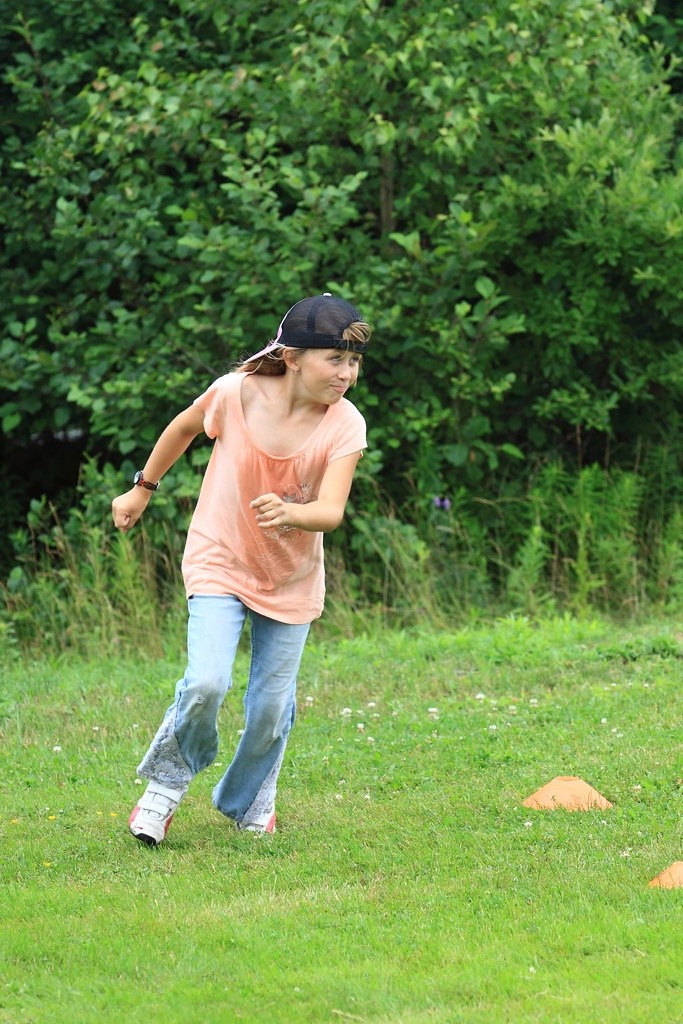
[242,291,367,366]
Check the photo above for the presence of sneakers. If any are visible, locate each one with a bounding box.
[235,801,276,838]
[127,779,190,843]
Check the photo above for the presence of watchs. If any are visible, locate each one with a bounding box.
[132,471,161,491]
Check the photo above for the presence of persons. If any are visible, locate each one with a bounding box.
[112,290,369,848]
